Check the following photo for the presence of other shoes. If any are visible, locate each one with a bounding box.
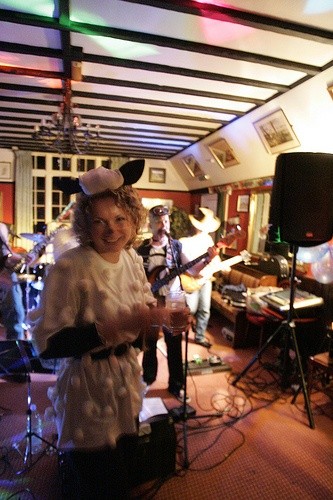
[196,340,212,348]
[167,388,191,404]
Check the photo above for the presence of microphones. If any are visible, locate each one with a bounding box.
[161,227,173,241]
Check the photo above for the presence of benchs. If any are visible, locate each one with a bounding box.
[211,263,278,350]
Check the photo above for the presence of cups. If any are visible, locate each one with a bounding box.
[165,291,188,334]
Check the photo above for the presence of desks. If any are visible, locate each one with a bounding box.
[262,307,321,374]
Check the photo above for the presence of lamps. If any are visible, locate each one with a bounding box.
[32,78,102,155]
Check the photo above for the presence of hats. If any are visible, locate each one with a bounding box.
[149,206,169,217]
[189,207,221,232]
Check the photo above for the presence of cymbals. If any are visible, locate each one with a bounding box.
[21,232,53,244]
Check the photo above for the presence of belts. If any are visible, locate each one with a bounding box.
[75,344,131,360]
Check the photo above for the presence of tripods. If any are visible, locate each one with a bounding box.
[0,267,65,475]
[163,242,226,472]
[232,246,316,429]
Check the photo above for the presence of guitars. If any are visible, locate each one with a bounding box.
[146,225,246,310]
[179,250,252,294]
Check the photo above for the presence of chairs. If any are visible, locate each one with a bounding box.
[304,323,333,420]
[240,287,272,352]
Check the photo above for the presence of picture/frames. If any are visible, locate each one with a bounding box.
[252,107,300,156]
[207,138,240,169]
[180,153,206,178]
[0,162,12,179]
[149,167,166,183]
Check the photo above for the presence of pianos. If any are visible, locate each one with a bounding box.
[259,286,323,311]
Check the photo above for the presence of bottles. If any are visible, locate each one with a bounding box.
[26,405,43,454]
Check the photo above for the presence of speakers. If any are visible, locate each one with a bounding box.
[268,152,333,248]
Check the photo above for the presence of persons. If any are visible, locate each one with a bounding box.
[0,206,230,404]
[27,159,196,500]
